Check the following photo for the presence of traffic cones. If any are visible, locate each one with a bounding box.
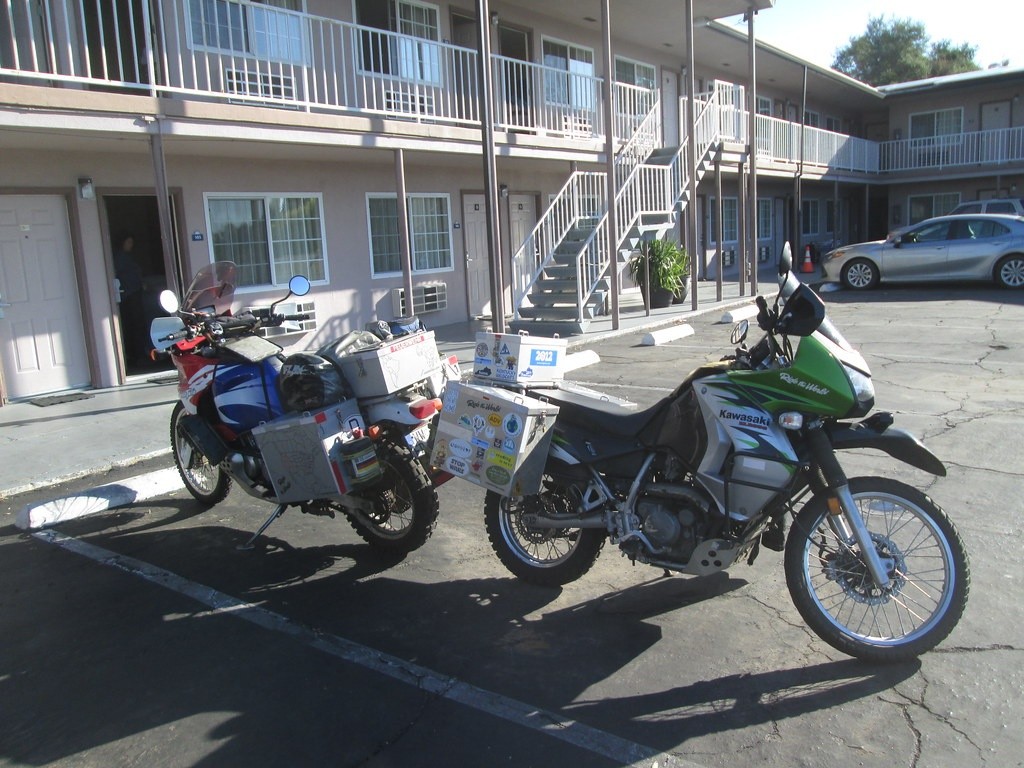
[798,245,817,273]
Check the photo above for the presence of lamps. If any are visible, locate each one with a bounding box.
[78,178,94,200]
[500,184,508,199]
[1012,184,1017,192]
[894,205,903,224]
[894,128,902,141]
[1014,93,1021,104]
[490,13,500,26]
[682,65,687,76]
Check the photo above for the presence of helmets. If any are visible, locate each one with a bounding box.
[280,353,353,411]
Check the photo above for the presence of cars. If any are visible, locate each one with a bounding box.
[821,212,1024,291]
[907,198,1024,235]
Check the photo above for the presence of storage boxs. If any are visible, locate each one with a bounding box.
[250,399,366,504]
[430,380,561,497]
[337,331,443,397]
[427,355,463,397]
[473,331,567,382]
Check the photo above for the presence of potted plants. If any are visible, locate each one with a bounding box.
[629,240,680,308]
[671,246,690,304]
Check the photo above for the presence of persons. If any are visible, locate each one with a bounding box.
[112,231,148,367]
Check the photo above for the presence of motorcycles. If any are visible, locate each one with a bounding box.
[149,261,445,552]
[469,237,971,665]
